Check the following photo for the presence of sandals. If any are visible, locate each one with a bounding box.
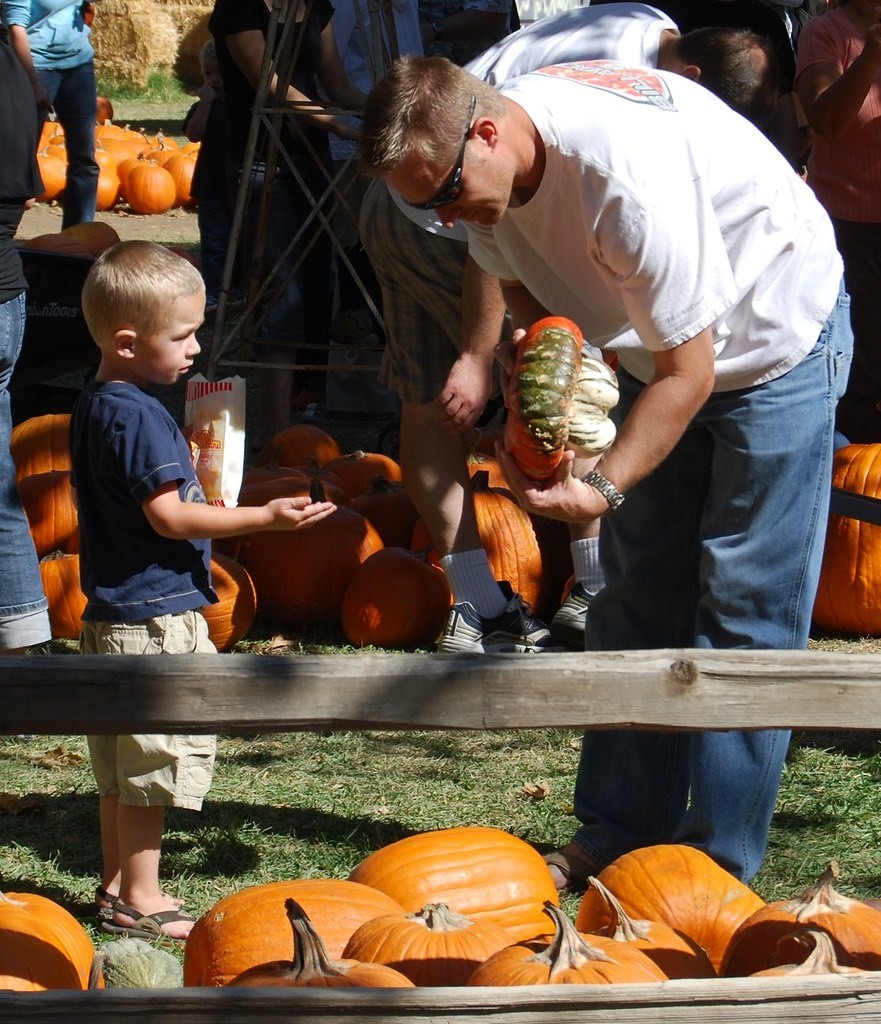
[100,902,194,944]
[95,885,186,920]
[541,849,597,897]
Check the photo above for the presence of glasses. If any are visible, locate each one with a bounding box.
[402,96,476,212]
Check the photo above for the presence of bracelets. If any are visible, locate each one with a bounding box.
[582,470,625,514]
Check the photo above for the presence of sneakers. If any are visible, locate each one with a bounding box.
[551,582,604,647]
[436,580,552,657]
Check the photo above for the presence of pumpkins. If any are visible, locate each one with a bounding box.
[14,317,622,653]
[810,442,881,638]
[0,824,880,993]
[33,119,207,214]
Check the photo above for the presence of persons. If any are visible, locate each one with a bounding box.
[360,54,853,878]
[190,0,524,457]
[363,0,775,652]
[590,0,881,446]
[68,241,338,944]
[0,36,52,654]
[0,0,100,231]
